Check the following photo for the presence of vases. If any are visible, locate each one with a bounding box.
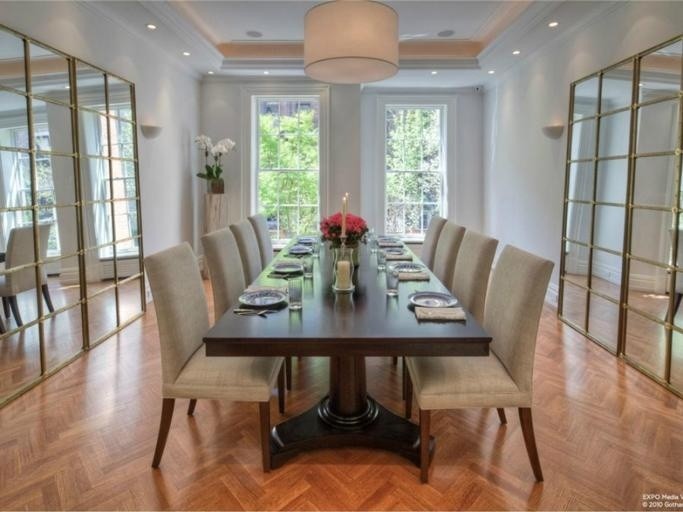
[214,180,223,195]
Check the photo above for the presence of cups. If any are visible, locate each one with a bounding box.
[288,310,304,335]
[332,247,354,289]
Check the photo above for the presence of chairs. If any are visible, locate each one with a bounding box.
[142,209,554,483]
[0,222,53,327]
[664,227,683,327]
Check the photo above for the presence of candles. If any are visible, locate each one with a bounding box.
[340,198,345,236]
[342,193,348,234]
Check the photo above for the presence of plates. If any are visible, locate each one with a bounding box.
[238,236,320,306]
[366,226,459,308]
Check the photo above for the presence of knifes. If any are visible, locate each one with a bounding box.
[233,307,278,313]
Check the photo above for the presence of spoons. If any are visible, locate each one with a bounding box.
[236,309,267,316]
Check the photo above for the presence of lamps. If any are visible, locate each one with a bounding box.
[301,0,399,88]
[541,124,565,140]
[139,125,162,139]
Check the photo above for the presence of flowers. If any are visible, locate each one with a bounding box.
[191,134,236,178]
